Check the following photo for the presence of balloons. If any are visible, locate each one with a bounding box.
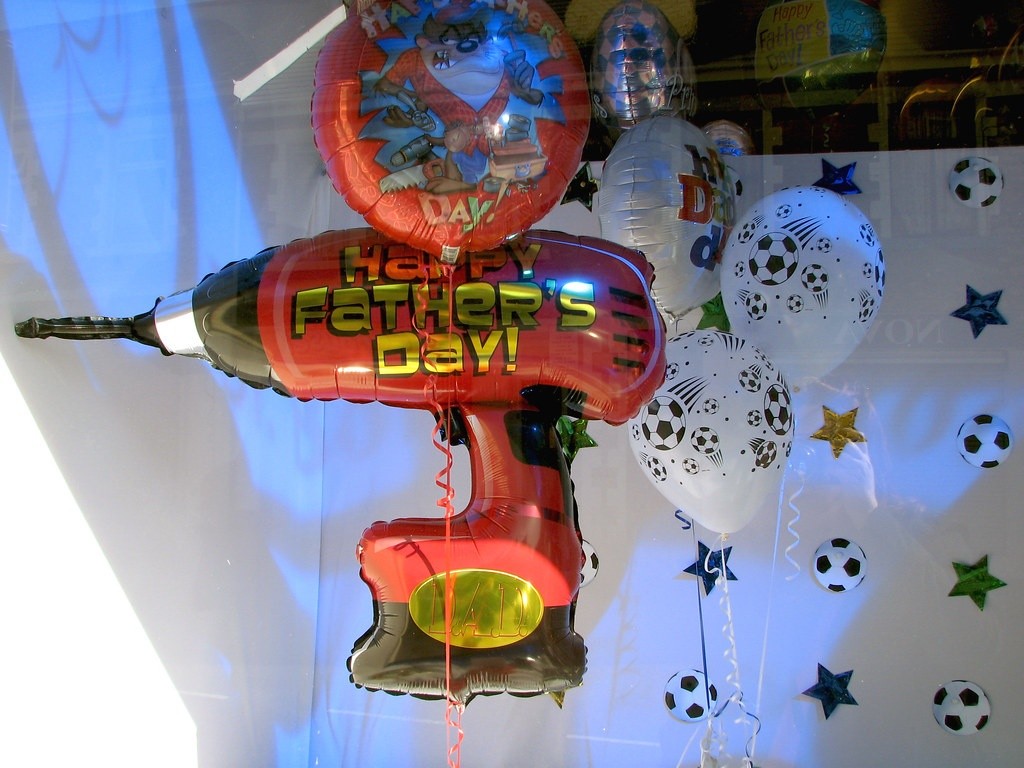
[591,0,702,132]
[312,1,592,262]
[753,0,888,126]
[704,120,756,157]
[598,117,739,319]
[627,329,796,541]
[720,185,887,389]
[14,227,667,698]
